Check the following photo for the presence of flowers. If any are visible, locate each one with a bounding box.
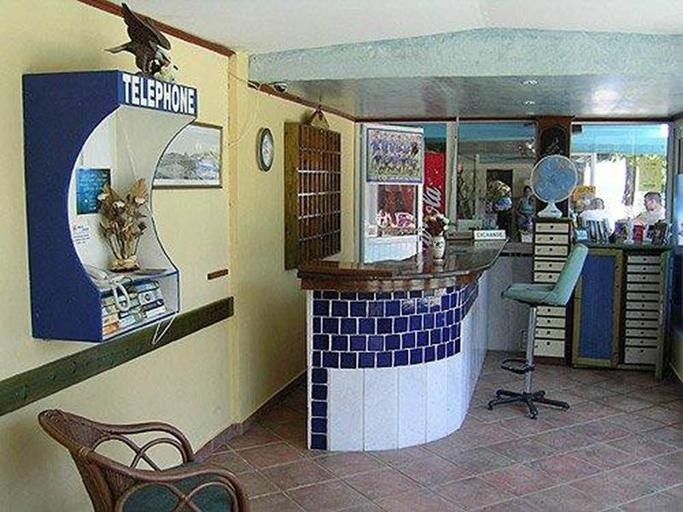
[97,177,149,260]
[422,213,450,236]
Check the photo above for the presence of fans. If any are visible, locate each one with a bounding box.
[530,154,578,218]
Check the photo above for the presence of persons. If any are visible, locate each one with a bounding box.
[636,191,669,228]
[577,197,608,230]
[493,191,514,242]
[513,185,537,230]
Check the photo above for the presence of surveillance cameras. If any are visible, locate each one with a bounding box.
[274,82,287,95]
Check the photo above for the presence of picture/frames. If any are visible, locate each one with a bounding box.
[151,121,223,189]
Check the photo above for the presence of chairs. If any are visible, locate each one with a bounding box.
[38,408,249,512]
[488,243,589,419]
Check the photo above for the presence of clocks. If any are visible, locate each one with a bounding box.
[256,128,274,172]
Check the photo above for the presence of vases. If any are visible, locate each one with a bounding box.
[110,255,140,272]
[432,234,446,259]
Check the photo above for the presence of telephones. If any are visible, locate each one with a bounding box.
[83,264,113,287]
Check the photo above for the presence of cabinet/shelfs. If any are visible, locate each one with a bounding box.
[22,70,197,343]
[532,218,683,383]
